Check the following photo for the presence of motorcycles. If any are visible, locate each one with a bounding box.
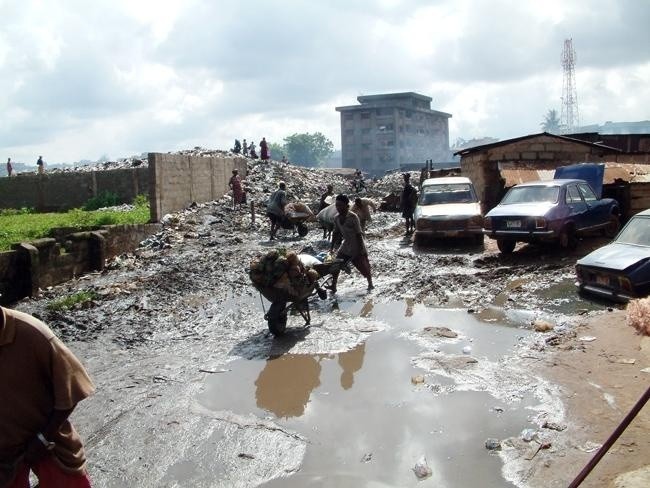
[354,178,365,192]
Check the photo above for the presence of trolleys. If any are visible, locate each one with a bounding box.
[268,212,312,237]
[246,246,355,337]
[316,220,335,241]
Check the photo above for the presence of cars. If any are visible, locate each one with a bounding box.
[412,177,485,246]
[575,206,650,305]
[482,162,622,253]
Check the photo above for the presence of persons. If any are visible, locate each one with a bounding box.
[324,194,374,291]
[355,198,372,232]
[281,156,288,164]
[37,156,45,174]
[266,181,289,241]
[353,169,365,185]
[402,173,417,236]
[318,185,334,241]
[229,169,243,211]
[7,158,12,177]
[233,138,271,162]
[0,305,96,488]
